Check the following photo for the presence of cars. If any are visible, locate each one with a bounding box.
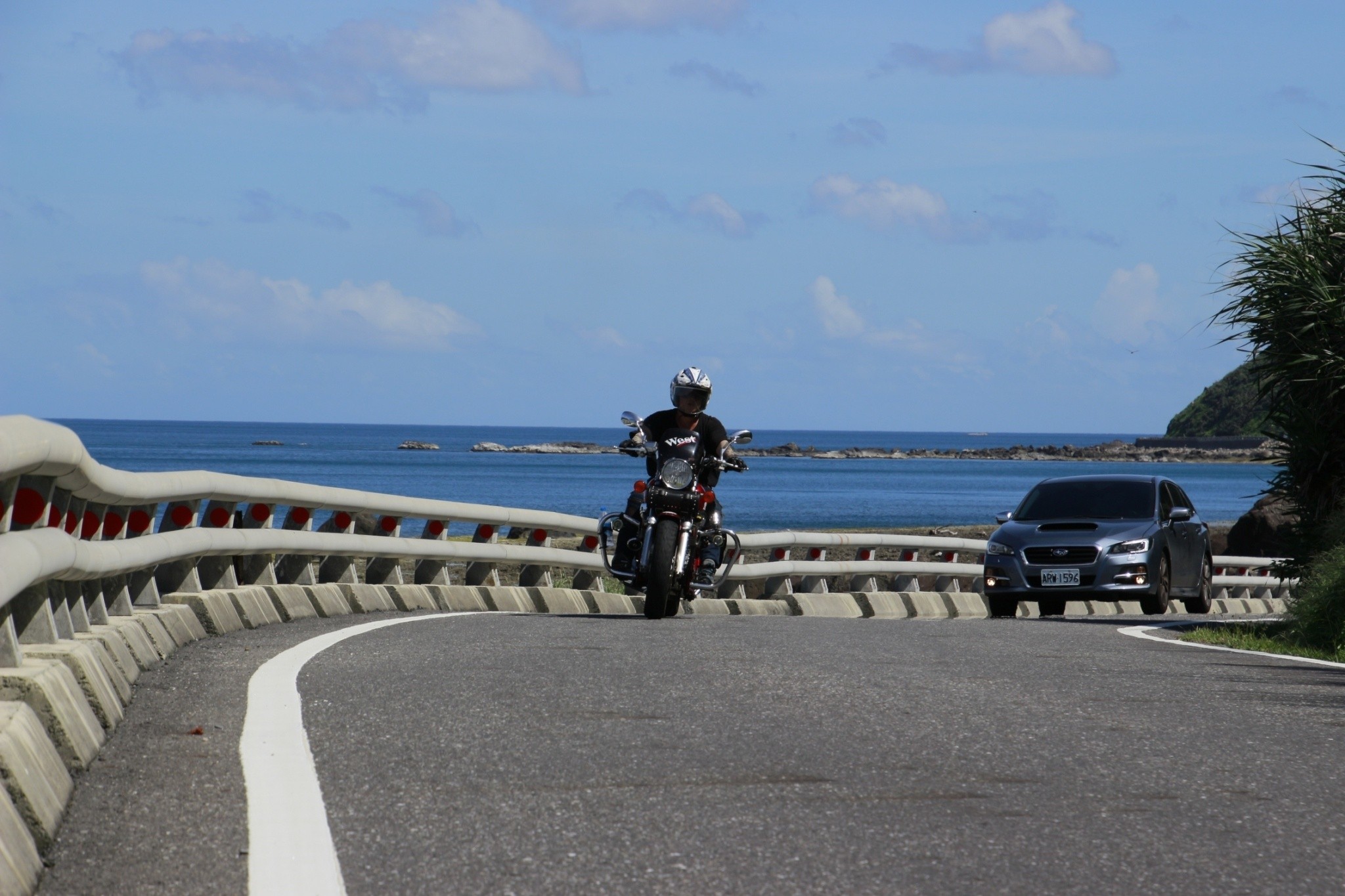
[982,474,1215,619]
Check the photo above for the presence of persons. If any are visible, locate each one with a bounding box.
[611,367,747,592]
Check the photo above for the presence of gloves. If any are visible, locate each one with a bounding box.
[724,457,747,473]
[619,440,639,457]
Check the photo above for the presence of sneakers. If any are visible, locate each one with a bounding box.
[699,563,717,585]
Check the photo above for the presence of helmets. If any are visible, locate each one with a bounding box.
[670,367,712,415]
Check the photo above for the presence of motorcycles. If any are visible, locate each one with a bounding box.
[597,411,754,620]
